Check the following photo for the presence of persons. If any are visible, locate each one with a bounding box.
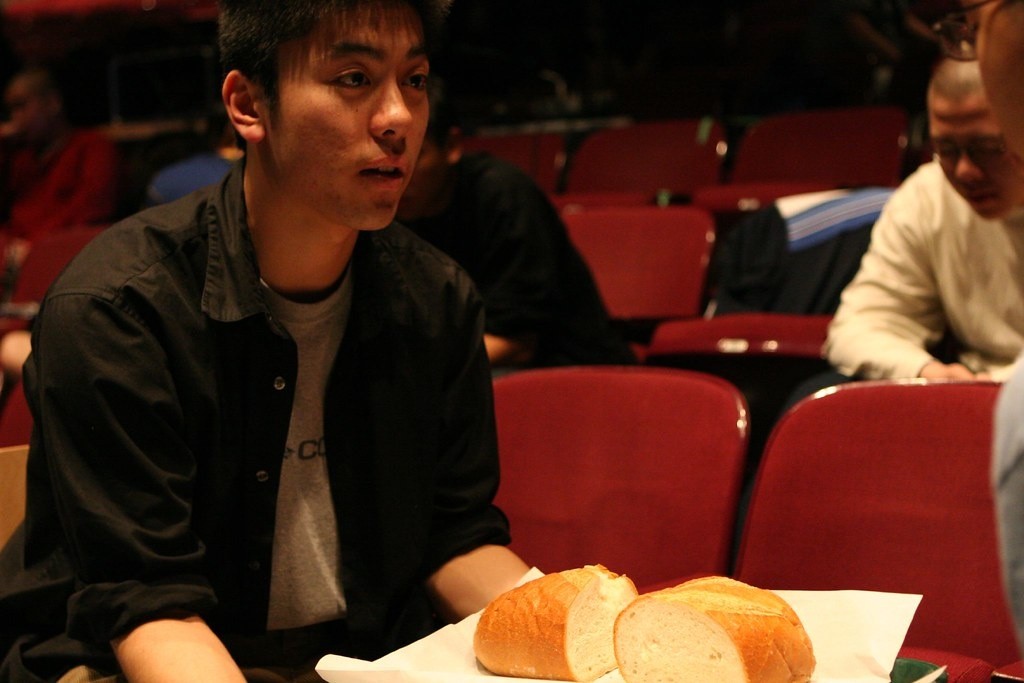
[0,0,663,364]
[732,0,1024,630]
[0,0,530,683]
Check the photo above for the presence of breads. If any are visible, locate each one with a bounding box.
[474,564,638,683]
[612,577,816,683]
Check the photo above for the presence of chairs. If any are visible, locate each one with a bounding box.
[0,104,1024,683]
